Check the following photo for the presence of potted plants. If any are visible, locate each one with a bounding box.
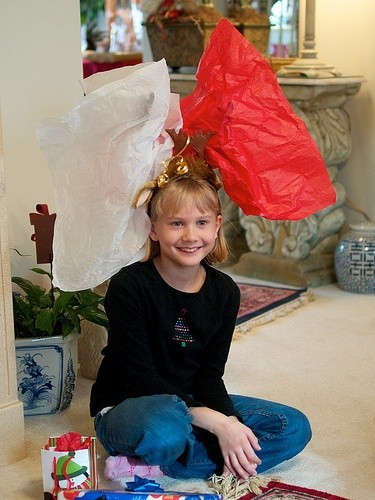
[11,260,108,417]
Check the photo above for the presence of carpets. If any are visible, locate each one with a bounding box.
[233,277,311,341]
[235,479,355,500]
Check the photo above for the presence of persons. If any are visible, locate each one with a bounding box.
[89,162,312,478]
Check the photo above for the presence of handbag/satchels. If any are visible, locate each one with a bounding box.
[40,434,97,500]
